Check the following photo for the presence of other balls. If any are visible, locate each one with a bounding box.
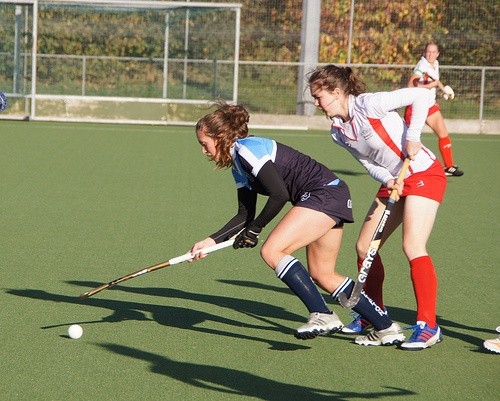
[68,324,83,340]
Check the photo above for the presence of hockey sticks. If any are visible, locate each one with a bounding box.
[337,157,411,310]
[80,238,235,299]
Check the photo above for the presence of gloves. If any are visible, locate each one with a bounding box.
[233,230,259,250]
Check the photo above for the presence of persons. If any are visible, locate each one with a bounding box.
[403,40,464,176]
[307,64,446,351]
[185,96,406,346]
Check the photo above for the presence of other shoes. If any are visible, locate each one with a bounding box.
[483,336,500,354]
[496,326,500,333]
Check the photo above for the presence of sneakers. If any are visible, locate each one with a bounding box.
[341,305,388,334]
[354,322,406,346]
[443,167,464,177]
[401,321,444,350]
[293,311,346,340]
